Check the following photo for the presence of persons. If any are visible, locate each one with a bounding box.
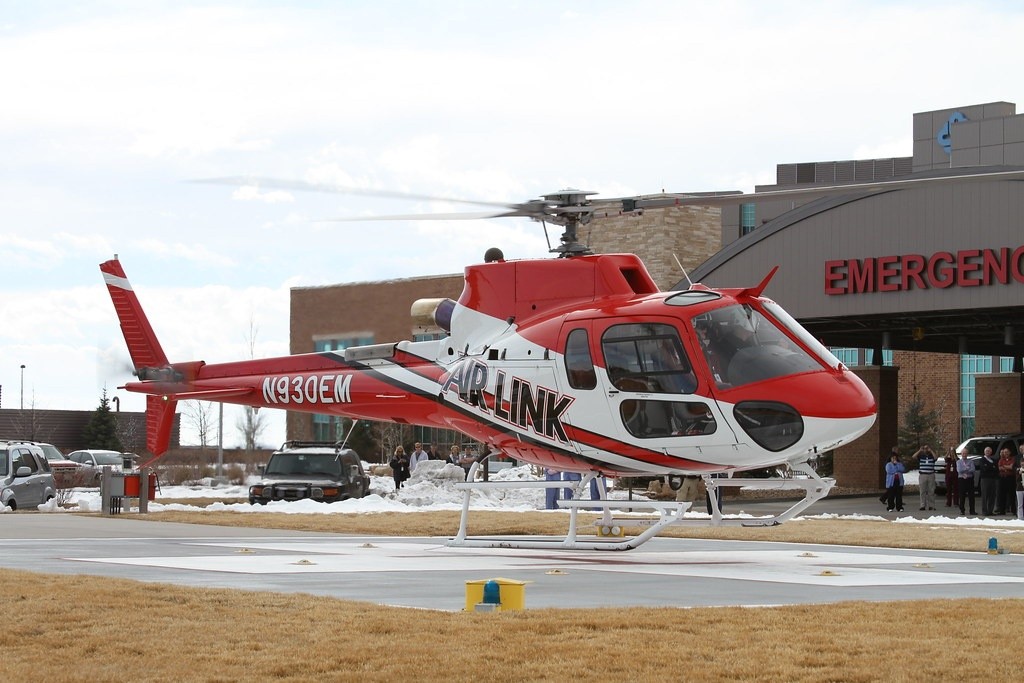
[912,444,938,510]
[427,441,440,460]
[980,447,997,517]
[991,445,1024,519]
[714,308,759,382]
[590,476,607,511]
[957,448,978,515]
[676,475,700,513]
[389,446,410,492]
[410,443,428,475]
[706,473,722,515]
[945,447,960,508]
[671,316,725,415]
[447,445,461,467]
[546,470,562,509]
[879,446,906,506]
[563,472,582,509]
[885,454,904,512]
[459,445,476,481]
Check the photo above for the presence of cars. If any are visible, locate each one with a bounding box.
[68,450,140,482]
[374,462,393,477]
[477,453,524,472]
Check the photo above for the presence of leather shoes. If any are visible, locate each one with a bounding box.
[879,498,887,505]
[902,502,906,505]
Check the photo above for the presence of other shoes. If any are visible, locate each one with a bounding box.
[982,513,988,516]
[994,510,997,512]
[998,513,1006,515]
[954,504,958,508]
[959,513,965,515]
[944,504,952,507]
[988,513,996,516]
[970,513,978,515]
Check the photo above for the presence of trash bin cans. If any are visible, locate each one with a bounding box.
[100,472,125,498]
[125,474,141,497]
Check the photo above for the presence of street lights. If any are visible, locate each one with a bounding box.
[21,365,25,409]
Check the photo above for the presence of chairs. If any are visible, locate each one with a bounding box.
[650,347,706,421]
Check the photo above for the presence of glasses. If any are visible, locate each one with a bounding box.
[398,451,403,453]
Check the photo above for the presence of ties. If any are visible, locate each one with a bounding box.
[962,459,968,479]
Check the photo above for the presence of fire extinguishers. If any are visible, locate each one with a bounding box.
[148,471,162,500]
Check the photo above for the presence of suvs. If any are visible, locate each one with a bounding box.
[0,440,57,511]
[933,432,1024,496]
[32,442,84,487]
[249,440,370,505]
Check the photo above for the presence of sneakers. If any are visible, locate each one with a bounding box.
[929,506,936,510]
[889,507,893,512]
[920,507,925,511]
[900,508,904,512]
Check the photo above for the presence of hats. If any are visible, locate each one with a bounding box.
[431,441,437,446]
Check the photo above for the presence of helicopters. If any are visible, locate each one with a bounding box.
[99,170,1024,552]
